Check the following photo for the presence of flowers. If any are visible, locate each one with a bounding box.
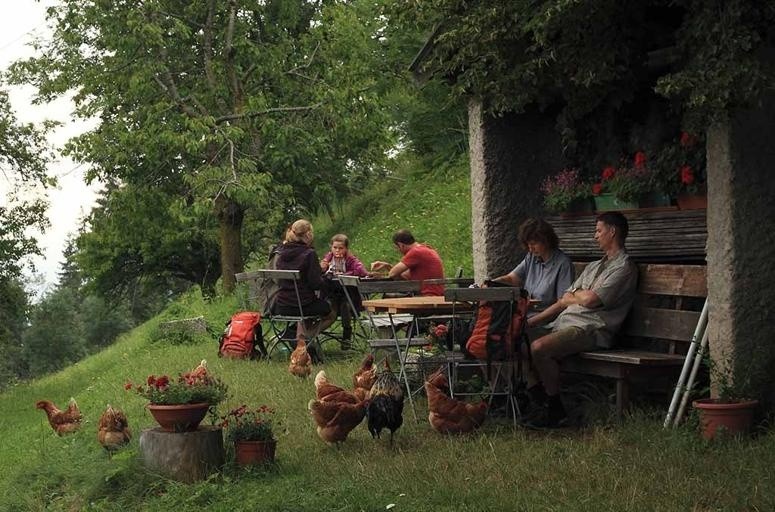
[595,150,659,201]
[123,364,229,404]
[653,133,712,191]
[220,403,293,443]
[540,166,593,212]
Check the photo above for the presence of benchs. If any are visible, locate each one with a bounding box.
[556,260,709,425]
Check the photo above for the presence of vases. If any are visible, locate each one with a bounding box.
[235,439,279,466]
[150,403,208,431]
[594,191,639,211]
[556,201,585,217]
[675,194,706,212]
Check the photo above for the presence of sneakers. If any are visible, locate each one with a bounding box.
[483,391,567,430]
[342,326,352,350]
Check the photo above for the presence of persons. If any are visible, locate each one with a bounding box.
[371,229,447,312]
[269,219,339,361]
[516,211,639,431]
[480,216,575,424]
[310,234,367,350]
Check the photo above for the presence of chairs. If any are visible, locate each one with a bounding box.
[320,270,361,354]
[359,280,421,384]
[440,286,525,434]
[255,267,323,363]
[236,270,277,360]
[338,272,387,361]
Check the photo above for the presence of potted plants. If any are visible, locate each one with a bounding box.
[693,344,769,445]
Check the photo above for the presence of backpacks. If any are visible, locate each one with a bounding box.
[218,311,271,360]
[460,280,530,361]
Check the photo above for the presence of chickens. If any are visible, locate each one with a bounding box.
[314,370,361,404]
[353,354,377,402]
[290,333,312,384]
[424,380,488,434]
[307,399,370,452]
[428,373,449,390]
[35,397,82,438]
[368,356,404,447]
[98,405,131,459]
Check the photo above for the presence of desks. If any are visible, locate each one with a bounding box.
[376,296,538,416]
